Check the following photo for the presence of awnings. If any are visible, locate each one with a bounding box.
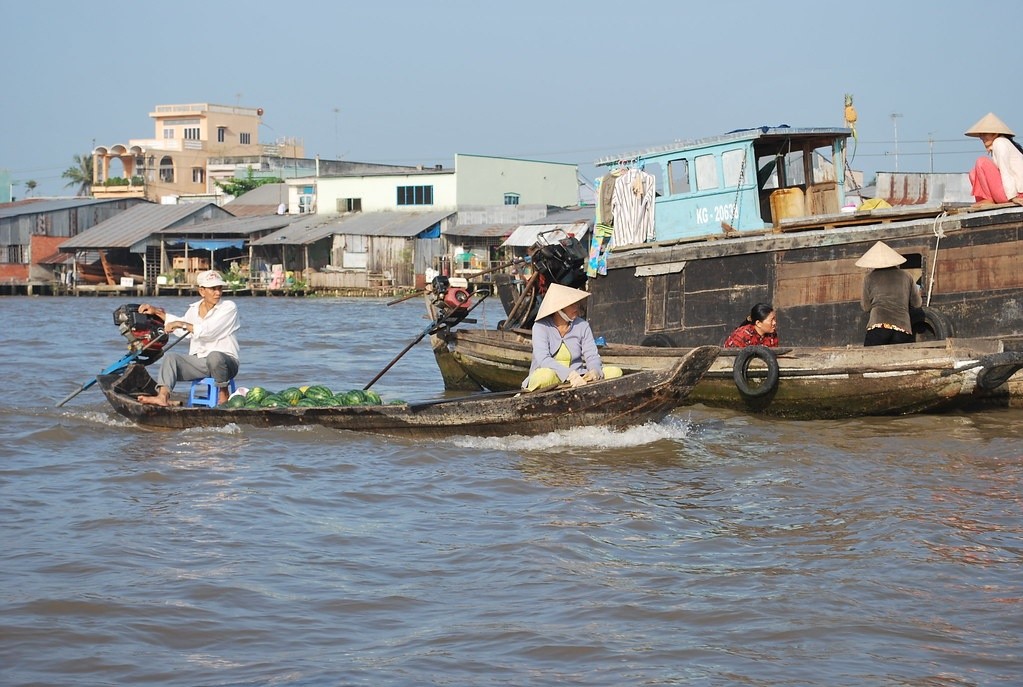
[153,214,334,245]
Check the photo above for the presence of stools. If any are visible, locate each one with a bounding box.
[187,377,236,409]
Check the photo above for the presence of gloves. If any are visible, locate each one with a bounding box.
[566,370,587,388]
[582,369,601,382]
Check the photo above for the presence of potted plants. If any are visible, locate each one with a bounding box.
[157,271,175,286]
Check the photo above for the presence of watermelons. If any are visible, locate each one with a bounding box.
[219,385,411,408]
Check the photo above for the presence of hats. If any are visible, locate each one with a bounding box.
[854,241,908,269]
[535,283,592,321]
[964,112,1016,138]
[197,270,228,288]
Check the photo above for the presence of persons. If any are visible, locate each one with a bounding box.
[136,270,241,407]
[855,241,922,347]
[964,113,1023,208]
[521,282,623,392]
[721,303,778,348]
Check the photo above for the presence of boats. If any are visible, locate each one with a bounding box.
[492,123,1023,340]
[425,272,1023,413]
[95,345,720,431]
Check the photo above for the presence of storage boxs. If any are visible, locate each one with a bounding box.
[173,258,209,284]
